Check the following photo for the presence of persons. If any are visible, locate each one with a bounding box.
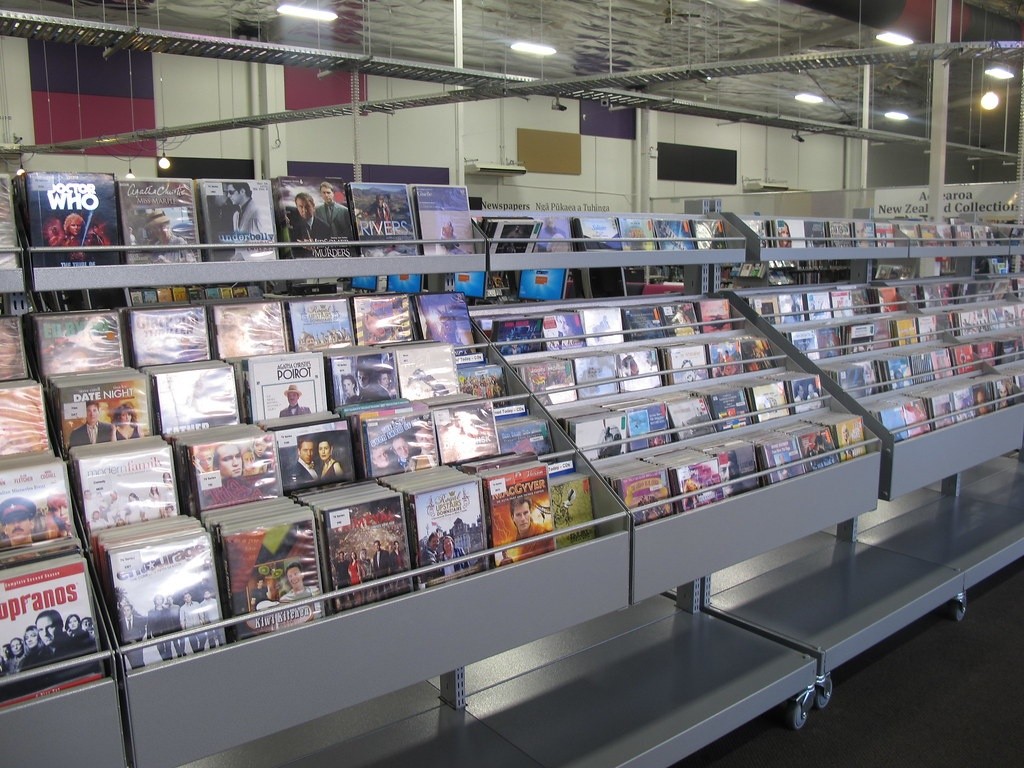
[0,178,1023,683]
